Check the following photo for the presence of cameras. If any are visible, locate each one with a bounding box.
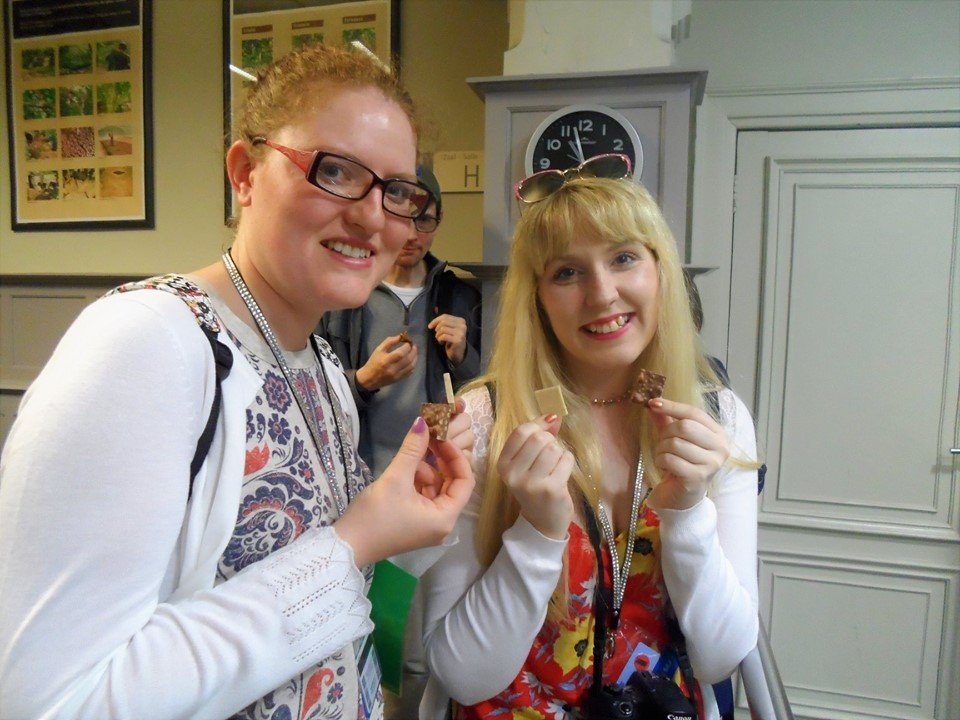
[583,670,697,720]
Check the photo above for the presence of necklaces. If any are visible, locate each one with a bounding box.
[591,383,634,407]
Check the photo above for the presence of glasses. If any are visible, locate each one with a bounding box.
[514,153,632,221]
[413,216,440,234]
[250,137,433,219]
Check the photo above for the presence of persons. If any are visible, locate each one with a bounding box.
[0,44,478,720]
[420,152,760,720]
[315,163,482,679]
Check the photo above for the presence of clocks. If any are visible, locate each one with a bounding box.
[524,103,643,183]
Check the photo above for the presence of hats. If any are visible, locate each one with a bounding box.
[415,164,441,217]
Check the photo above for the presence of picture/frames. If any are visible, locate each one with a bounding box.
[2,0,156,234]
[220,0,401,228]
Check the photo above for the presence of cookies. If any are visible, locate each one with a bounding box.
[421,403,453,442]
[534,385,568,418]
[631,369,666,408]
[443,372,456,413]
